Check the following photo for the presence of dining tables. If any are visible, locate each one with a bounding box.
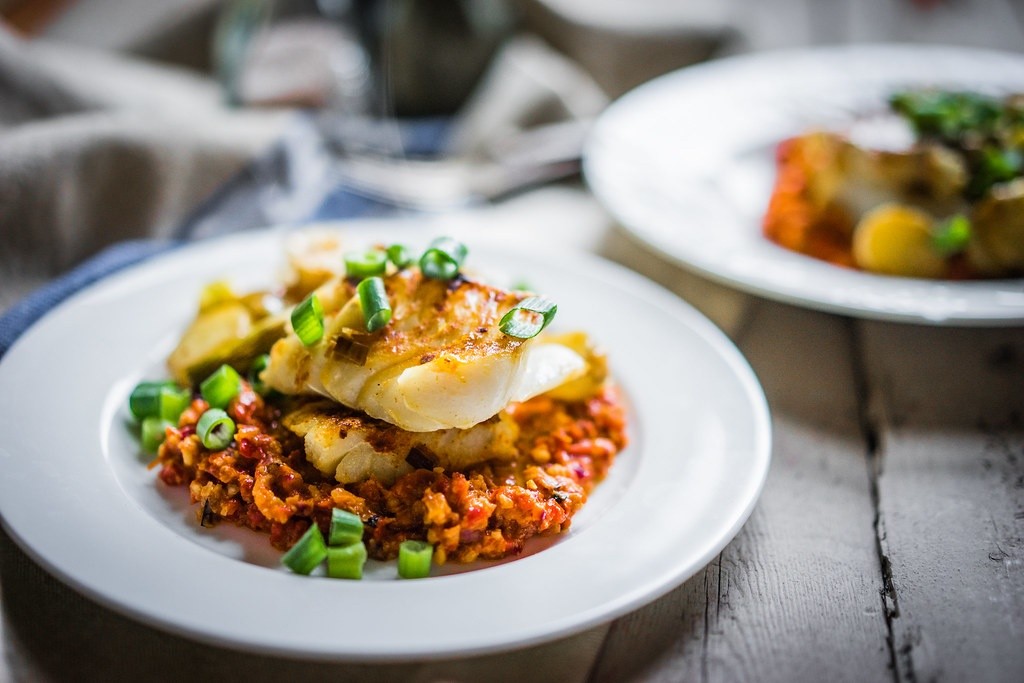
[0,178,1023,683]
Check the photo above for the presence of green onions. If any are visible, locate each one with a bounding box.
[129,234,559,578]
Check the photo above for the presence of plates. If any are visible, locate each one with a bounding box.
[581,39,1023,331]
[2,213,772,663]
